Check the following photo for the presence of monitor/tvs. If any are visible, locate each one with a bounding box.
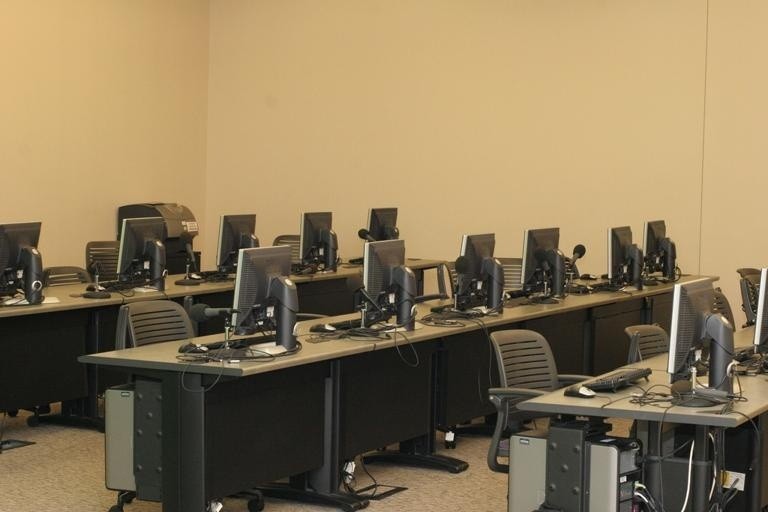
[216,214,260,270]
[457,233,506,315]
[364,239,417,333]
[367,208,399,242]
[0,223,44,306]
[518,228,566,300]
[607,226,644,292]
[299,212,338,274]
[752,268,768,359]
[643,220,676,283]
[231,245,300,351]
[116,217,167,293]
[667,278,736,408]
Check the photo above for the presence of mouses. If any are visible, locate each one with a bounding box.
[309,324,337,333]
[178,343,209,354]
[564,385,596,399]
[580,274,597,281]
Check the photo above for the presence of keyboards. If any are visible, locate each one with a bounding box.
[328,318,362,332]
[581,369,652,394]
[348,258,365,266]
[568,282,609,294]
[86,280,147,293]
[202,334,277,349]
[430,304,456,314]
[507,290,524,299]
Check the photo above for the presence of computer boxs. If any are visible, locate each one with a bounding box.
[105,383,136,492]
[507,429,643,512]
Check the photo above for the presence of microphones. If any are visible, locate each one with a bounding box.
[177,231,195,260]
[345,274,385,315]
[568,245,586,270]
[358,230,376,242]
[188,304,239,323]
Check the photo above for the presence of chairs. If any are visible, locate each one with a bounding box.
[0,233,766,512]
